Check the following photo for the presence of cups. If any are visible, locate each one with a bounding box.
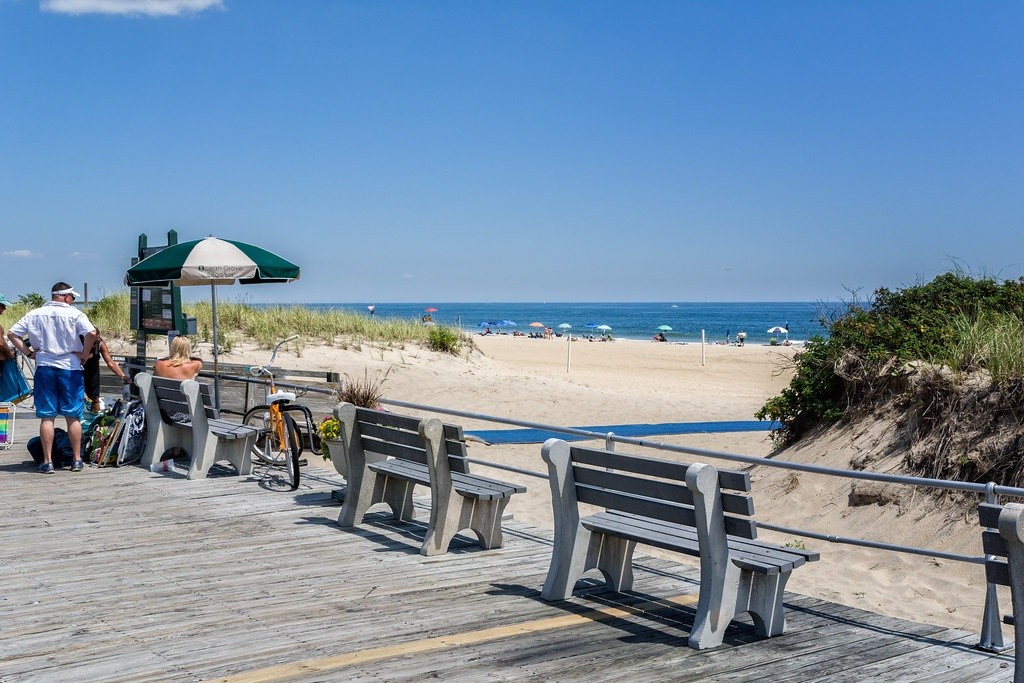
[150,459,174,472]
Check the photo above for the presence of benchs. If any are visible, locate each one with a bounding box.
[133,372,262,481]
[541,437,821,649]
[976,502,1024,683]
[333,402,527,556]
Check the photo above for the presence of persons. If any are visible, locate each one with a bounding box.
[423,315,432,323]
[79,325,131,417]
[543,326,553,340]
[7,282,97,473]
[0,293,14,398]
[151,337,203,382]
[736,331,747,348]
[368,304,375,317]
[589,334,613,342]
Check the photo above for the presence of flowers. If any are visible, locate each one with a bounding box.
[319,415,342,439]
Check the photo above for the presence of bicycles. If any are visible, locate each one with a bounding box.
[242,334,304,489]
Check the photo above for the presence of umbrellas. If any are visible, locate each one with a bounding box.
[477,320,516,334]
[584,323,613,338]
[656,324,672,341]
[767,326,788,346]
[529,321,544,334]
[424,307,438,323]
[124,234,301,419]
[557,323,573,335]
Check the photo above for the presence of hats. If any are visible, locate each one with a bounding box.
[51,286,80,297]
[0,293,14,307]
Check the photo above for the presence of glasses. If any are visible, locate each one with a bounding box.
[68,294,75,300]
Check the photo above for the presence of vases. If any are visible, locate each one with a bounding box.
[325,440,387,481]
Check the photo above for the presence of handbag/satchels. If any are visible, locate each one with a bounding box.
[27,428,74,469]
[83,399,126,464]
